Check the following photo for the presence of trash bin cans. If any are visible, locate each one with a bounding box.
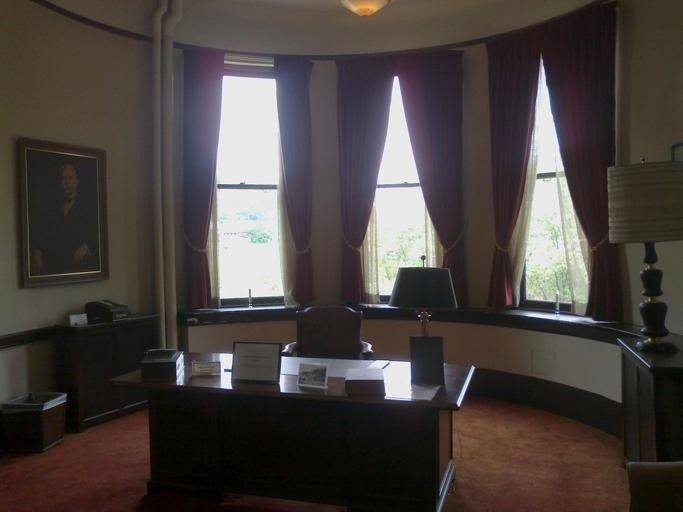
[0,391,68,453]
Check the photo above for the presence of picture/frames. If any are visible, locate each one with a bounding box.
[296,362,329,389]
[14,134,111,289]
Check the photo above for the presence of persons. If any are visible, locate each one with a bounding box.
[30,161,99,276]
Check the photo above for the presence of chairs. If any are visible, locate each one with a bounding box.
[282,304,373,360]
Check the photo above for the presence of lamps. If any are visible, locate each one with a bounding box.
[341,1,390,16]
[387,256,459,386]
[607,158,682,355]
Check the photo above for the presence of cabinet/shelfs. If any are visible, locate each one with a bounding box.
[616,335,682,469]
[52,314,159,432]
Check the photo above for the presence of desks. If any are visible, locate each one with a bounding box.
[109,353,477,511]
